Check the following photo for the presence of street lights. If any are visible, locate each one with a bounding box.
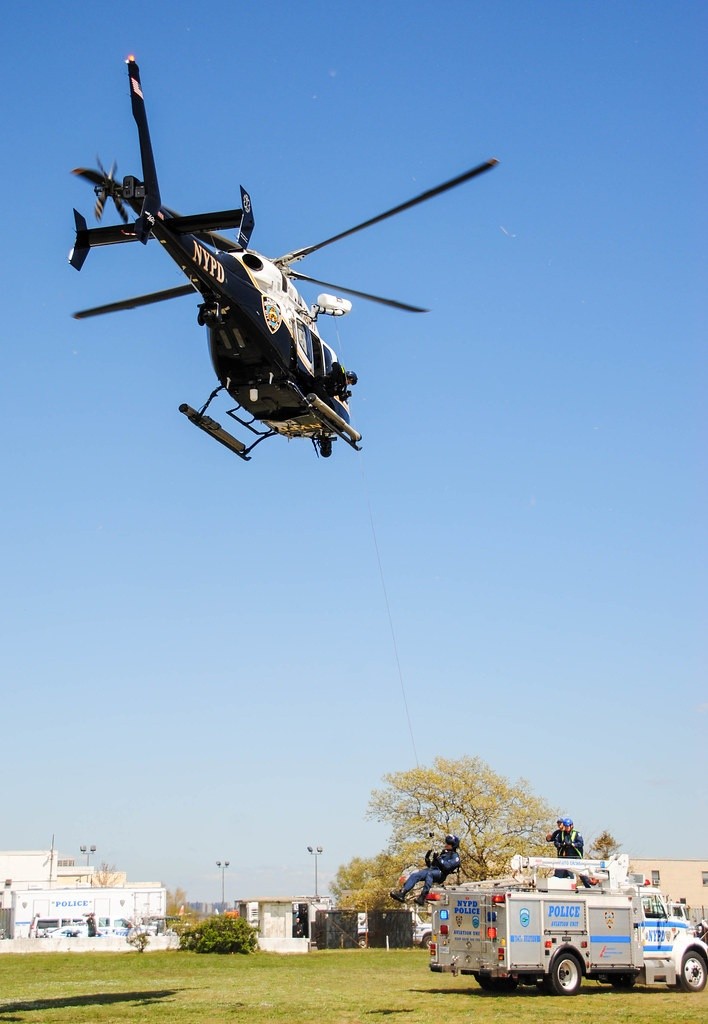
[306,846,324,903]
[79,845,96,866]
[215,860,230,914]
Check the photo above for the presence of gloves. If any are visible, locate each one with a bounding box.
[426,850,432,859]
[433,851,439,859]
[560,839,573,848]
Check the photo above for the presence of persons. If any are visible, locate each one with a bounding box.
[546,818,591,888]
[390,835,460,905]
[296,917,304,937]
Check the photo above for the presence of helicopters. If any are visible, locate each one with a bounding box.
[66,53,502,462]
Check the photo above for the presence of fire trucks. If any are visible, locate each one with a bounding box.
[422,853,708,996]
[357,911,433,949]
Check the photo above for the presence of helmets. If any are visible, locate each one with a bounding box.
[557,817,564,823]
[563,818,573,826]
[445,835,459,848]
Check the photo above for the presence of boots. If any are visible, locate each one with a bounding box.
[390,888,407,903]
[414,892,426,906]
